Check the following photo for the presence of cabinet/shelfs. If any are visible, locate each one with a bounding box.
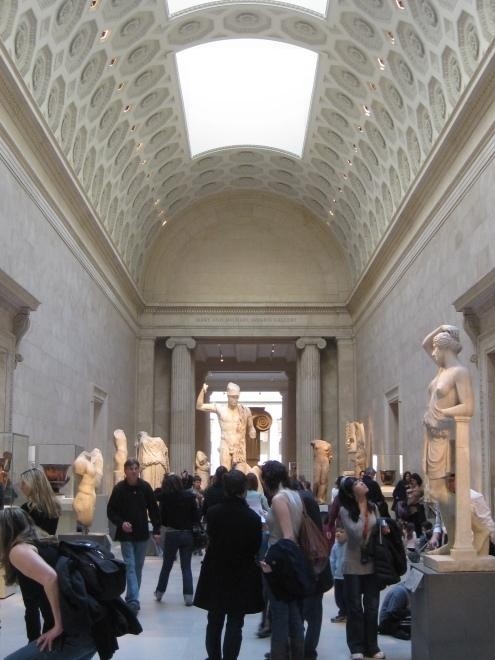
[371,453,405,519]
[28,443,92,543]
[0,432,30,600]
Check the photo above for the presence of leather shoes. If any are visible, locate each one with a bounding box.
[393,629,410,639]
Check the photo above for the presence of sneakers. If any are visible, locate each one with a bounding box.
[128,602,140,610]
[375,651,384,659]
[331,617,346,624]
[257,629,270,637]
[351,653,363,660]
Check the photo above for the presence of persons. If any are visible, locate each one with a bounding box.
[311,439,333,504]
[378,471,434,638]
[422,325,492,556]
[154,471,205,607]
[18,468,63,643]
[107,458,162,611]
[192,459,391,660]
[0,507,97,660]
[196,382,257,474]
[430,473,495,556]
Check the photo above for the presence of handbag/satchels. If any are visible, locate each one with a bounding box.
[297,513,330,576]
[193,526,208,550]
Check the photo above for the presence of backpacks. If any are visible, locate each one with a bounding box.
[57,537,128,603]
